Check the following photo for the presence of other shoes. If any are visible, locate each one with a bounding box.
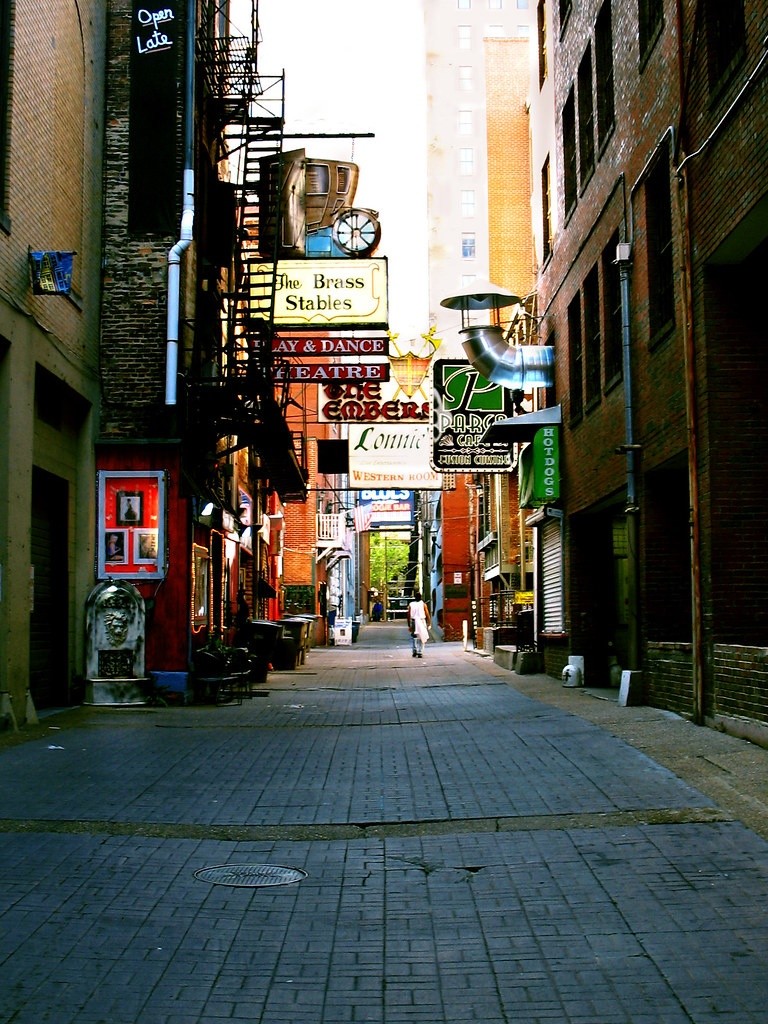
[413,650,417,657]
[418,654,422,658]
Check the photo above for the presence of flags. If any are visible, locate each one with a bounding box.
[354,503,372,533]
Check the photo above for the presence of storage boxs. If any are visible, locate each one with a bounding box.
[153,671,187,693]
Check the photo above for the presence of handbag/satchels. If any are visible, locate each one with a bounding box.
[411,619,417,637]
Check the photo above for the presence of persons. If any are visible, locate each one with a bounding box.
[407,593,431,657]
[373,600,382,621]
[107,535,124,560]
[124,498,136,519]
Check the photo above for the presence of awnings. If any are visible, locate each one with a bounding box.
[478,403,561,443]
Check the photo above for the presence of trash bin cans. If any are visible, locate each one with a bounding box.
[276,613,317,670]
[242,621,283,682]
[352,622,360,643]
